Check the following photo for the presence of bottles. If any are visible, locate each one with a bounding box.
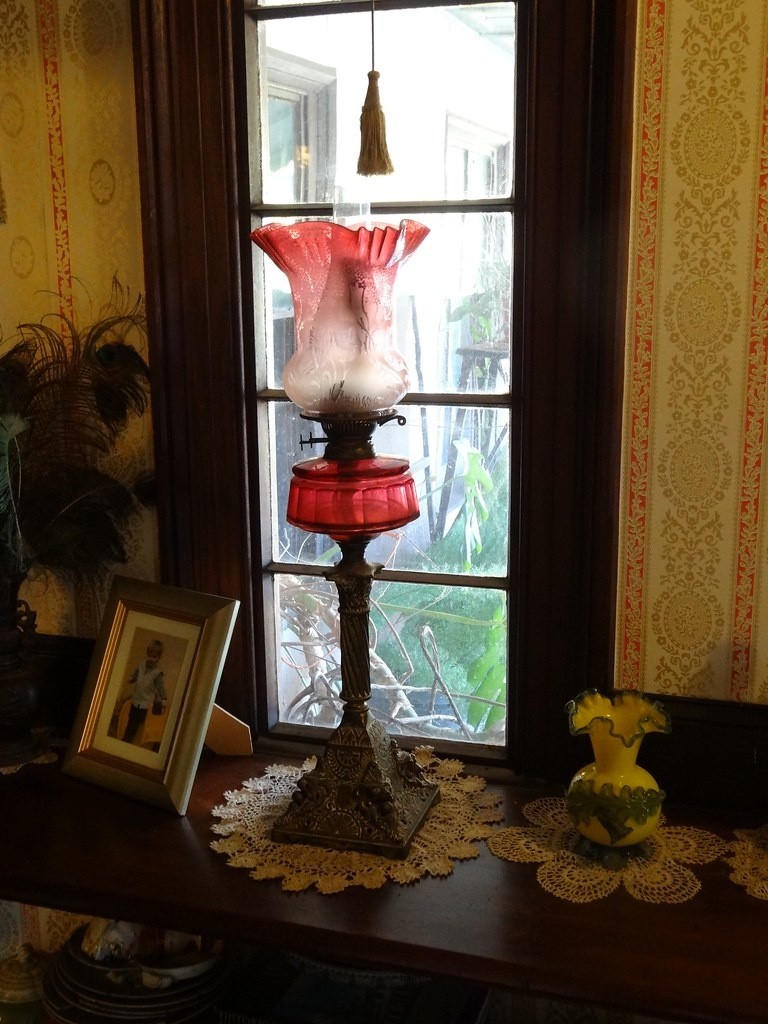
[565,688,669,848]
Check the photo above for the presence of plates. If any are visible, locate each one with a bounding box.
[40,923,236,1023]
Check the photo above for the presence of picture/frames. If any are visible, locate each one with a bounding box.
[60,575,254,816]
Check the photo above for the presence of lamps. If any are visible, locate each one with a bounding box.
[250,218,438,858]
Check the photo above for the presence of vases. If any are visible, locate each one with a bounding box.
[566,688,671,872]
